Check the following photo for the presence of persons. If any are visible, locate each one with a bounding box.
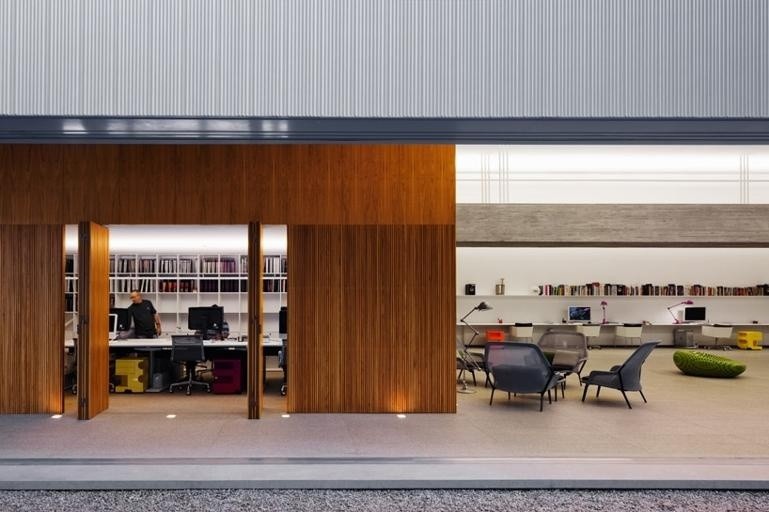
[194,304,229,340]
[127,290,162,338]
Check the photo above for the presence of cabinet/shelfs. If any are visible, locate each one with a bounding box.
[63,252,288,332]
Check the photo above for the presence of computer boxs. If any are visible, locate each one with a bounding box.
[674,330,694,346]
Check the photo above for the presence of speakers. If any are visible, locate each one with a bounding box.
[466,284,475,295]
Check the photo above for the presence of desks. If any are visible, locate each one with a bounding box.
[63,339,287,393]
[456,323,769,344]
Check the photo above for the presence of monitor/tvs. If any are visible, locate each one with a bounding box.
[683,305,707,323]
[279,310,288,334]
[107,313,118,341]
[568,306,592,323]
[188,306,224,340]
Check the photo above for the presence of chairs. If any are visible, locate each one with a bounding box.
[168,334,212,396]
[456,329,664,413]
[576,323,601,350]
[509,323,533,343]
[614,323,643,348]
[701,324,734,351]
[276,339,288,396]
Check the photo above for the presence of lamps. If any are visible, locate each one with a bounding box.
[600,300,609,324]
[668,300,693,324]
[457,301,493,394]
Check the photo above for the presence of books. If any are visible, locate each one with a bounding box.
[538,283,769,297]
[65,254,287,313]
